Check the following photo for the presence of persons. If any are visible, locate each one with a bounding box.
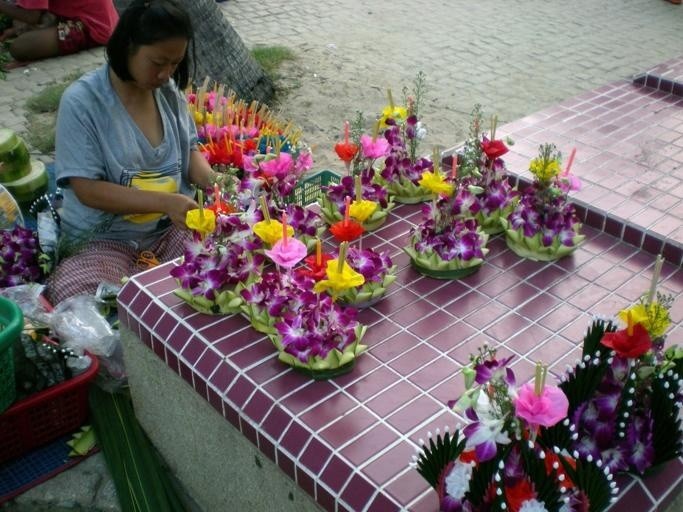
[42,1,230,326]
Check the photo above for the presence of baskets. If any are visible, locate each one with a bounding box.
[282,170,344,210]
[0,298,99,464]
[0,294,23,412]
